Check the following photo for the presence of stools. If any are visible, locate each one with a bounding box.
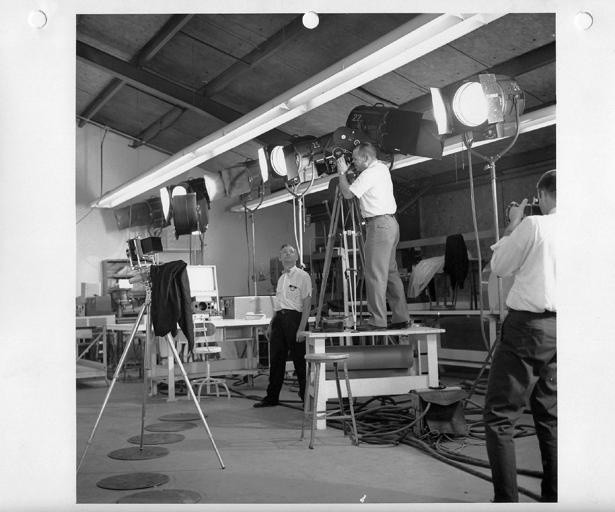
[298,352,360,451]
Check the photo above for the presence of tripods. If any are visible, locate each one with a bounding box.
[76,270,225,473]
[315,184,365,331]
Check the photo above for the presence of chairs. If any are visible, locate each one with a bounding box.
[187,322,231,403]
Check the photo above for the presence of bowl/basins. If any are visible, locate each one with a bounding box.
[241,314,265,321]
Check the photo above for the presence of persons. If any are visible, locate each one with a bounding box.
[335,140,411,332]
[483,168,557,503]
[254,242,313,408]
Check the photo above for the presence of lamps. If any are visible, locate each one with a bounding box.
[159,137,316,239]
[429,72,529,164]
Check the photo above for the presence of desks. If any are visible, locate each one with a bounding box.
[302,326,446,430]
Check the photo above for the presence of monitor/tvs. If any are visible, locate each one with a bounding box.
[185,265,218,298]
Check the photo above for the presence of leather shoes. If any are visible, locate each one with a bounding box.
[357,323,413,331]
[253,399,279,408]
